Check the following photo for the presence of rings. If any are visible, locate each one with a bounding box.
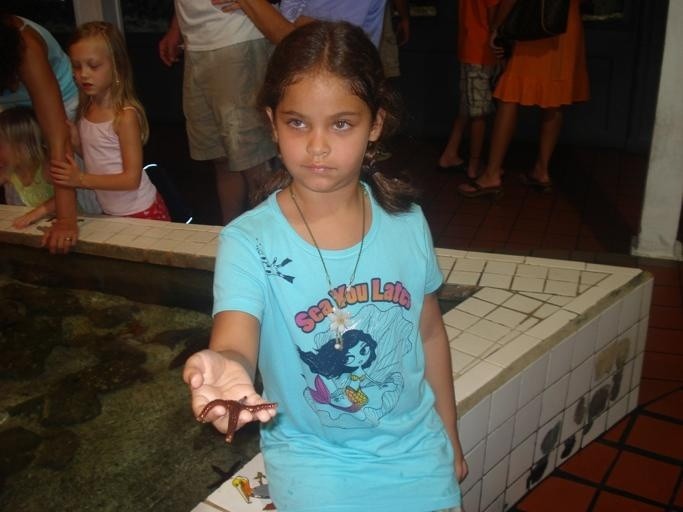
[64,237,71,241]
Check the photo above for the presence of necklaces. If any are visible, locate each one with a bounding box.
[287,181,367,350]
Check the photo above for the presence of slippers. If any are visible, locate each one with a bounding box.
[525,169,552,187]
[436,161,503,196]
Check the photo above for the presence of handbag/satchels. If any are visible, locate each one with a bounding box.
[499,1,567,40]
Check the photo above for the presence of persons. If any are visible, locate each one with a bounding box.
[180,17,469,512]
[155,0,590,255]
[47,19,172,222]
[0,104,82,231]
[0,6,103,256]
